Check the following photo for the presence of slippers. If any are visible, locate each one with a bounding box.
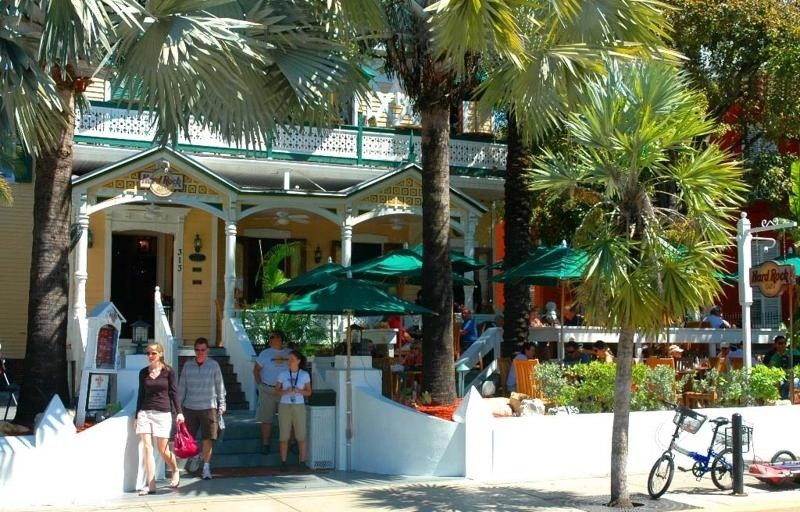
[170,471,180,488]
[139,487,156,496]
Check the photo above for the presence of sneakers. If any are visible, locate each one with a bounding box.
[189,454,203,472]
[202,470,212,479]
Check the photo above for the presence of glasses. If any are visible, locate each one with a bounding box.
[146,352,158,356]
[195,348,205,352]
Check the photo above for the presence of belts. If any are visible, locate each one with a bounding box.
[263,382,275,388]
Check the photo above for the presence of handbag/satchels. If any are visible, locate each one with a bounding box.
[172,420,200,458]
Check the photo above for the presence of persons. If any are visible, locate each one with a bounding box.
[506,343,535,397]
[253,330,292,455]
[275,350,312,473]
[133,343,184,492]
[178,338,225,479]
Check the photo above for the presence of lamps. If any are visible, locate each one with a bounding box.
[344,322,366,357]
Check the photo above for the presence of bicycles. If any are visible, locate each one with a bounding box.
[646,397,755,499]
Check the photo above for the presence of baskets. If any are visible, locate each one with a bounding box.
[673,411,702,434]
[715,423,753,446]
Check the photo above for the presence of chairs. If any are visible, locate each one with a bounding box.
[374,310,800,410]
